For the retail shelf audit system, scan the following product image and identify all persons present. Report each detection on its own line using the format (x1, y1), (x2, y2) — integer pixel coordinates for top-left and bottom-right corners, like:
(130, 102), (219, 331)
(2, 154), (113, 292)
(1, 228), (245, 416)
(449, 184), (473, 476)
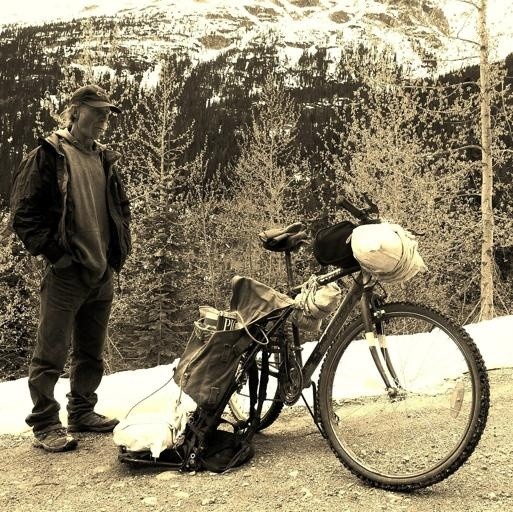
(10, 84), (131, 452)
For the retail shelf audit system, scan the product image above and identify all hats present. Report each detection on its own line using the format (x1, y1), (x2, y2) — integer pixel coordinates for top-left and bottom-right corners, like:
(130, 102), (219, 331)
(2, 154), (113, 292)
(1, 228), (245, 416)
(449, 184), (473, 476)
(70, 85), (122, 115)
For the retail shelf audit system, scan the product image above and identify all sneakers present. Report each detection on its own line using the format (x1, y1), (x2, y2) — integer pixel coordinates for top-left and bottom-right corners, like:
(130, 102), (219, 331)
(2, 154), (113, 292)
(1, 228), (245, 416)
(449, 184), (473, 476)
(67, 411), (120, 433)
(32, 427), (78, 452)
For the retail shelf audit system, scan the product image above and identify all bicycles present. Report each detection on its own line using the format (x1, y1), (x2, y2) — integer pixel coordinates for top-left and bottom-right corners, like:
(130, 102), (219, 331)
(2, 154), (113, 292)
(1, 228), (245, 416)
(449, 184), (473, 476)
(228, 192), (490, 492)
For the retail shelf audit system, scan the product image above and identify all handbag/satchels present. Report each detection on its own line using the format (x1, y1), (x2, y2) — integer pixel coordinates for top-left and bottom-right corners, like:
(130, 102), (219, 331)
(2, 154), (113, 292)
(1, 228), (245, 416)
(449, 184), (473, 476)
(170, 274), (296, 414)
